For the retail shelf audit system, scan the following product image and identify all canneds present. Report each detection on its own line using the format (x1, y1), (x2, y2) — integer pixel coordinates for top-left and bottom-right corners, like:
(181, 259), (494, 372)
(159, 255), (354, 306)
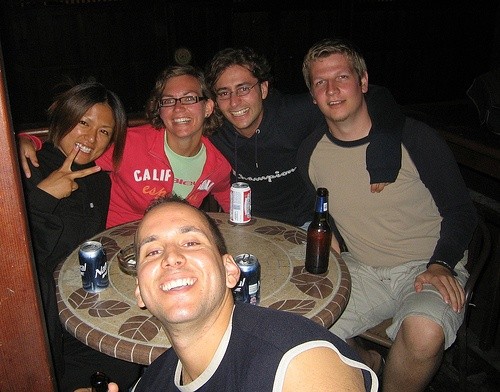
(231, 253), (260, 306)
(229, 182), (252, 223)
(78, 240), (109, 294)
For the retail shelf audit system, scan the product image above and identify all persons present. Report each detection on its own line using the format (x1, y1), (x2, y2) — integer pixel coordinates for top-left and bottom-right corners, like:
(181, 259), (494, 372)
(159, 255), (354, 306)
(22, 82), (128, 387)
(73, 193), (380, 392)
(296, 37), (476, 392)
(14, 66), (239, 229)
(206, 46), (402, 227)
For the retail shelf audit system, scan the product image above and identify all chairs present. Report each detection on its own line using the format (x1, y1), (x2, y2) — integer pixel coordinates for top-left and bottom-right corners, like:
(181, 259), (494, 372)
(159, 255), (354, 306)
(17, 115), (149, 156)
(359, 218), (488, 392)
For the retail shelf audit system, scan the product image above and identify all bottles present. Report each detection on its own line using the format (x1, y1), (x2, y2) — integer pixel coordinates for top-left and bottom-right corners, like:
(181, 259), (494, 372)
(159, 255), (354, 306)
(90, 372), (108, 392)
(305, 187), (332, 275)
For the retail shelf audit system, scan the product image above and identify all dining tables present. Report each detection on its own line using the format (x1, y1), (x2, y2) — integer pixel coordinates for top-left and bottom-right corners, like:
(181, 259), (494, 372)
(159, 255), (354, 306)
(52, 211), (352, 368)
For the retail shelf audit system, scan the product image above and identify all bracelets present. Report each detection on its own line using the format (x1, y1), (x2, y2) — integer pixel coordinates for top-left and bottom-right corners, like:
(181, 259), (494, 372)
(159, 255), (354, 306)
(421, 258), (458, 275)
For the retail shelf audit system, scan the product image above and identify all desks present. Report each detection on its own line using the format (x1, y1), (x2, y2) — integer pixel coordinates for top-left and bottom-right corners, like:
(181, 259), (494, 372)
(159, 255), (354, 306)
(420, 110), (500, 203)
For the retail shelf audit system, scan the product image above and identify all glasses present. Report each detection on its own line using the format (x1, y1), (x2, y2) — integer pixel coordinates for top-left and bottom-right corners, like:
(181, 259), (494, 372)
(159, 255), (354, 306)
(158, 96), (208, 108)
(216, 81), (259, 101)
